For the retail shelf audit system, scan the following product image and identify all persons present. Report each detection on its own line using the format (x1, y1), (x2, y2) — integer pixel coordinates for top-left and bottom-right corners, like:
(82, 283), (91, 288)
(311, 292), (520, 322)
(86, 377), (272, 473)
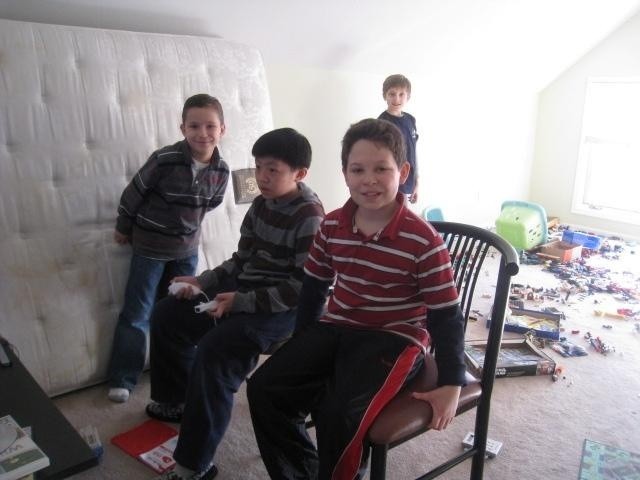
(142, 126), (328, 480)
(103, 91), (232, 403)
(245, 117), (469, 480)
(376, 72), (420, 219)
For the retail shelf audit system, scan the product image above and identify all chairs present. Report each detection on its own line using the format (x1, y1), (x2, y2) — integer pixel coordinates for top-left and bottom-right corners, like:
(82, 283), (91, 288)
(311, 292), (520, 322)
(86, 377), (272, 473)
(300, 216), (523, 480)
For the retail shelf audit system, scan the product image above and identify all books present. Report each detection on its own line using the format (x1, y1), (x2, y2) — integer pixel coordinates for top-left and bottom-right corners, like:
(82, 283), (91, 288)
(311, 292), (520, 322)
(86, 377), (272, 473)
(109, 416), (182, 475)
(579, 437), (639, 479)
(0, 413), (52, 479)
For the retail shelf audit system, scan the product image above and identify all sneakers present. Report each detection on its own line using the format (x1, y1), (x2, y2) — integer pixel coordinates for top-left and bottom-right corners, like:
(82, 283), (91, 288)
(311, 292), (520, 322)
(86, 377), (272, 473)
(147, 403), (184, 422)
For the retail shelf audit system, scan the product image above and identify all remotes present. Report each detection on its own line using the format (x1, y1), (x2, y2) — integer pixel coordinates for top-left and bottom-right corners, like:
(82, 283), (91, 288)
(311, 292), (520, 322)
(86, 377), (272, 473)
(462, 432), (502, 456)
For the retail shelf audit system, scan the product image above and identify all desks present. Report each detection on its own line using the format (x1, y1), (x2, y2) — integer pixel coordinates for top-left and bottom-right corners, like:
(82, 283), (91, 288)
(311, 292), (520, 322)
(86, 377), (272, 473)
(0, 339), (101, 479)
